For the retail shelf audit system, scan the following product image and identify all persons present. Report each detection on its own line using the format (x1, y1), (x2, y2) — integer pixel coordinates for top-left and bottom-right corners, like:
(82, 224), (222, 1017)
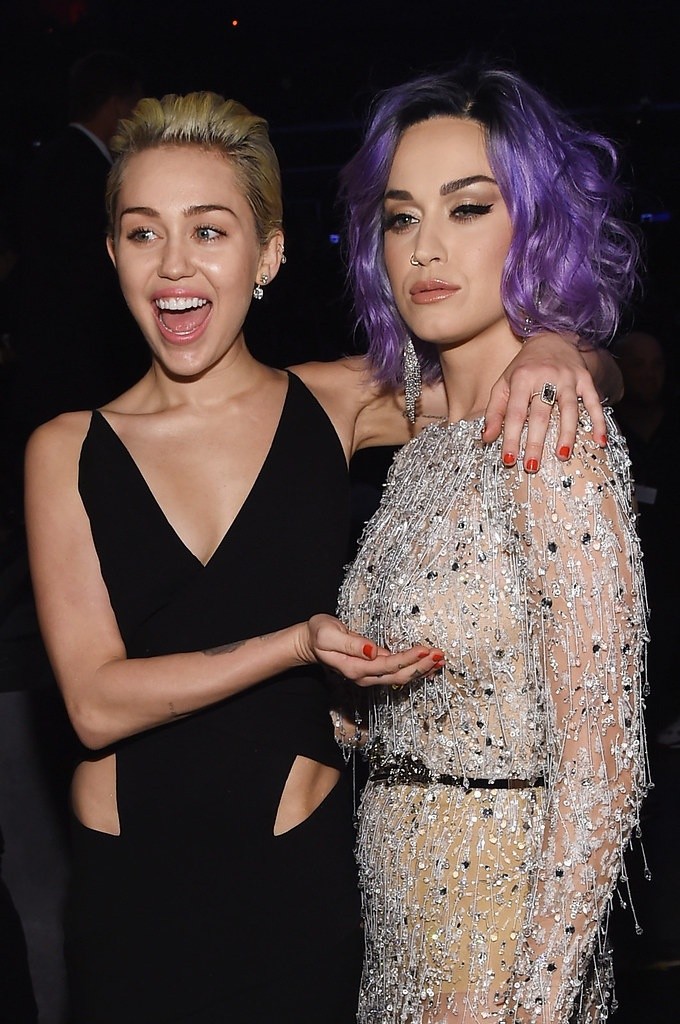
(337, 63), (648, 1024)
(24, 92), (631, 1023)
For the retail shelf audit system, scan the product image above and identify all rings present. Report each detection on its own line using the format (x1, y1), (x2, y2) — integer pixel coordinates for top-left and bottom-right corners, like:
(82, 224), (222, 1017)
(391, 683), (404, 691)
(530, 381), (557, 405)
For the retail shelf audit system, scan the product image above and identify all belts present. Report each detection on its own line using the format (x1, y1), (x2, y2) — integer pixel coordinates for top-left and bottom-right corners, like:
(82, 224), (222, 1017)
(366, 747), (550, 789)
